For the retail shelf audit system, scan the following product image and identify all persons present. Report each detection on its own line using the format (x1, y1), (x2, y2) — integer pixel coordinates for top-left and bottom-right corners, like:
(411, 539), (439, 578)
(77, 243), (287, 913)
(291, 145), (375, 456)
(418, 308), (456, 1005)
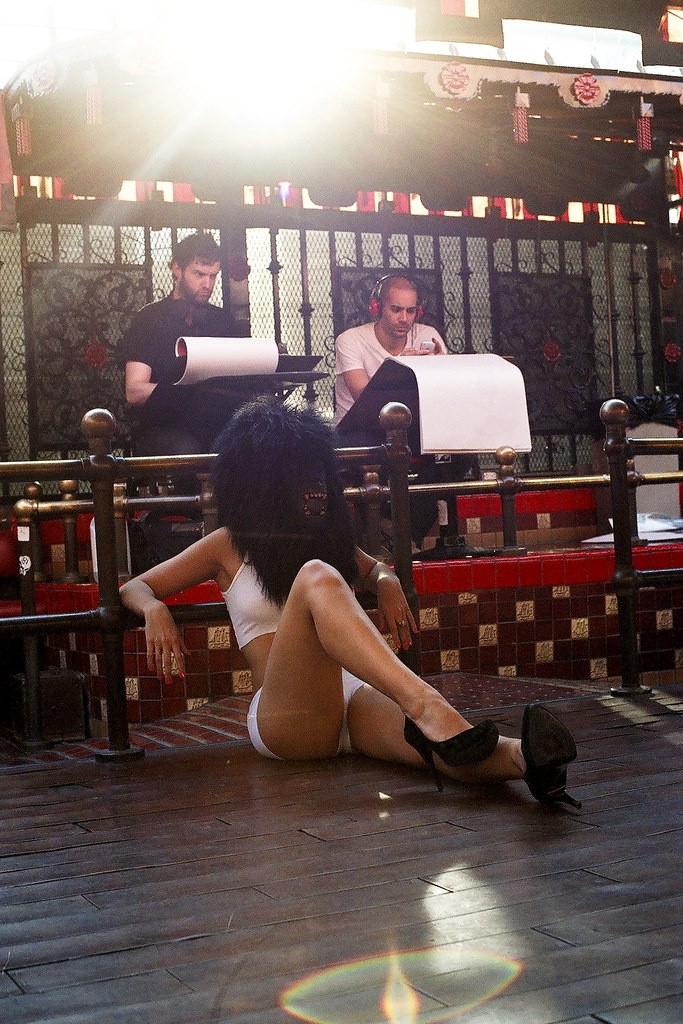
(331, 274), (479, 566)
(114, 234), (272, 482)
(115, 404), (580, 813)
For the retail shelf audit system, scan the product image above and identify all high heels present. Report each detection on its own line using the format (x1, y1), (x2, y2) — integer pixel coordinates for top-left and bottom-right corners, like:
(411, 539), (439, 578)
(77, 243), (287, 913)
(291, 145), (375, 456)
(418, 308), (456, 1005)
(402, 713), (501, 791)
(518, 699), (582, 812)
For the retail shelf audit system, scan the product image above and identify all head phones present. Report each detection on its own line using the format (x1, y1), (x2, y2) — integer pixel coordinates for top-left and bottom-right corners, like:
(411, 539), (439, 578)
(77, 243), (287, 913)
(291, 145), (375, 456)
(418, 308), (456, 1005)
(369, 274), (423, 320)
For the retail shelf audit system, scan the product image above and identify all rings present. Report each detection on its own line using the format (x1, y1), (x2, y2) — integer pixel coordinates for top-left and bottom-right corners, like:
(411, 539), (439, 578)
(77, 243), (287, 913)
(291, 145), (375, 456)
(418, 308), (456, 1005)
(397, 620), (405, 626)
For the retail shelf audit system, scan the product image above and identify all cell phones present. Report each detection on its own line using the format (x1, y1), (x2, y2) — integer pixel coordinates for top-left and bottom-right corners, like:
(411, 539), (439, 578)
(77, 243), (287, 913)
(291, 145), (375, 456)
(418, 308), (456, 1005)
(419, 342), (436, 353)
